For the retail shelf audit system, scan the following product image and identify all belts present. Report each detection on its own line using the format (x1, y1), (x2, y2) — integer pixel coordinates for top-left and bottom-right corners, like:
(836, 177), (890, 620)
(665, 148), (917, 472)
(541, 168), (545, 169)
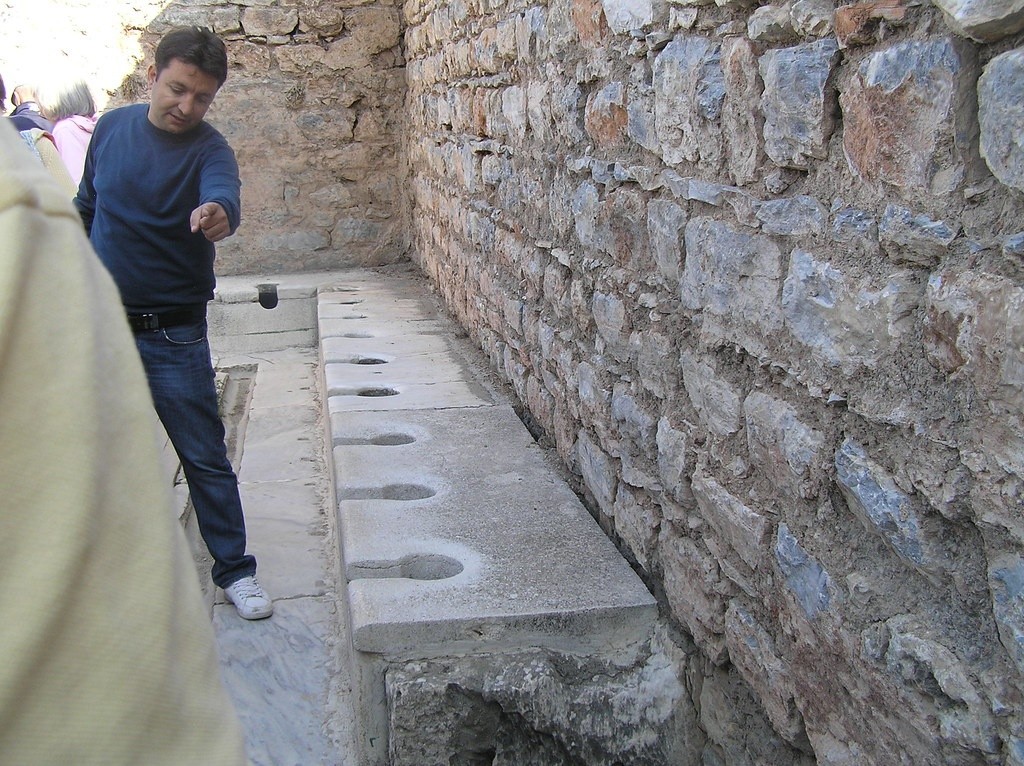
(126, 303), (207, 332)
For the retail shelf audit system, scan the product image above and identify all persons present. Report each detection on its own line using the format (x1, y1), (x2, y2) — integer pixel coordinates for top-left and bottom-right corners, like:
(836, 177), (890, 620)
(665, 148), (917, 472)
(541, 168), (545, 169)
(9, 82), (57, 134)
(0, 108), (238, 766)
(20, 127), (79, 199)
(76, 25), (272, 621)
(35, 63), (98, 188)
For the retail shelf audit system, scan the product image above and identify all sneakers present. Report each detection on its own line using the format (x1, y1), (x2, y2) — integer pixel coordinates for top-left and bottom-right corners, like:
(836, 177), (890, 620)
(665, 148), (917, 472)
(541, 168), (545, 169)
(224, 576), (273, 620)
